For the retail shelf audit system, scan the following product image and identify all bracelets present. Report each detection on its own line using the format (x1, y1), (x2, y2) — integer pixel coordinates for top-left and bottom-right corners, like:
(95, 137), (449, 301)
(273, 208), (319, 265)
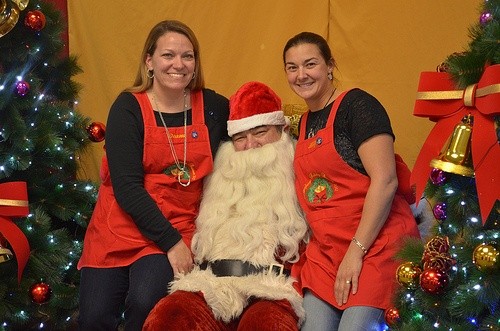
(352, 238), (367, 255)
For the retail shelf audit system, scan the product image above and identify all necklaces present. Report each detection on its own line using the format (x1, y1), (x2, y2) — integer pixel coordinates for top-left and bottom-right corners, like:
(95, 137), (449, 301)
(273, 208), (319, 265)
(151, 90), (191, 188)
(308, 89), (336, 140)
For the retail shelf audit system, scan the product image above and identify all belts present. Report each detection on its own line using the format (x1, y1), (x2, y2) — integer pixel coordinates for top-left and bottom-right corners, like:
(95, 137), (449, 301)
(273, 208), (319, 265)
(199, 259), (290, 277)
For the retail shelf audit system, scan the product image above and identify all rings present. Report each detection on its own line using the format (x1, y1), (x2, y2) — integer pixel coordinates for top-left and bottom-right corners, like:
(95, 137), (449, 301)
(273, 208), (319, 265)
(346, 281), (350, 283)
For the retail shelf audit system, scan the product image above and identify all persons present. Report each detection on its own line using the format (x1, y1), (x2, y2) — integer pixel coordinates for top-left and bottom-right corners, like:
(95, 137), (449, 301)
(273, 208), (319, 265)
(142, 83), (423, 331)
(283, 32), (421, 331)
(75, 20), (289, 331)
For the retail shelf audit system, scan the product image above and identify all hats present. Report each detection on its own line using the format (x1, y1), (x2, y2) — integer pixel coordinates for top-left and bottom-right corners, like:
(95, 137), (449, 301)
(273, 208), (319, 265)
(227, 81), (291, 137)
